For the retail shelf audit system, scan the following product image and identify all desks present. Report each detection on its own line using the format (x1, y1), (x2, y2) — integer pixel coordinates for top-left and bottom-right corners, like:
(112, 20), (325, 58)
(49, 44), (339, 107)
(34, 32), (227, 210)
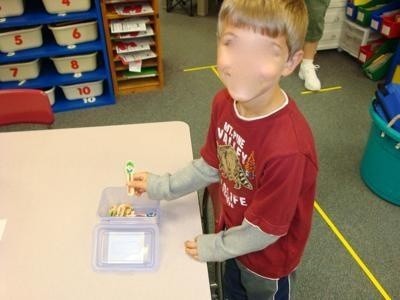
(2, 120), (214, 300)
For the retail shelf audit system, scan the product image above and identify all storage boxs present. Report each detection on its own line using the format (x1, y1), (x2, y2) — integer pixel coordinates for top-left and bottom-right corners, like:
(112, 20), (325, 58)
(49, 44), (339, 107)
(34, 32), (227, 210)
(90, 183), (164, 274)
(1, 1), (106, 109)
(340, 0), (400, 81)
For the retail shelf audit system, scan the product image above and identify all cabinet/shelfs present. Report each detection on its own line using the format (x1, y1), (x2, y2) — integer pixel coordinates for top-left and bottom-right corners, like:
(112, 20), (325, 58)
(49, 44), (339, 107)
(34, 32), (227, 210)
(1, 0), (116, 114)
(101, 0), (166, 96)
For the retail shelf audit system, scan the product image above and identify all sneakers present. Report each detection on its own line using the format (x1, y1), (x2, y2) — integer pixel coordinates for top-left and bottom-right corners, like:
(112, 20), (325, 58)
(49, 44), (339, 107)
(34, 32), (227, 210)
(298, 62), (321, 91)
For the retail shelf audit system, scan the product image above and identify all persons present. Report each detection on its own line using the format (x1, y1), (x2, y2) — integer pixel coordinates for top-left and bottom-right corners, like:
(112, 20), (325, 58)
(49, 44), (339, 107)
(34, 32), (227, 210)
(296, 0), (330, 93)
(127, 0), (320, 300)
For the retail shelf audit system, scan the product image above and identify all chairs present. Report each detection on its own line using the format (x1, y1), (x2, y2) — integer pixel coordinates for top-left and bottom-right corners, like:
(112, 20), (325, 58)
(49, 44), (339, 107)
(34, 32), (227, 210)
(1, 89), (57, 130)
